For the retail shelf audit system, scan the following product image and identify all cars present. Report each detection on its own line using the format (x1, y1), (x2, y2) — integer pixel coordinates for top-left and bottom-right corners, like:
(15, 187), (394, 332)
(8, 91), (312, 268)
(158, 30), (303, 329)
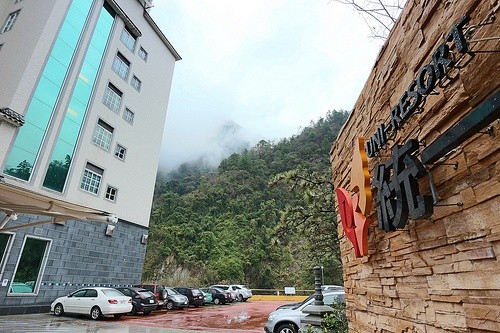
(200, 290), (213, 305)
(264, 292), (345, 333)
(164, 288), (189, 311)
(115, 287), (158, 316)
(233, 285), (252, 302)
(51, 286), (133, 321)
(10, 282), (34, 293)
(276, 285), (344, 311)
(205, 287), (232, 305)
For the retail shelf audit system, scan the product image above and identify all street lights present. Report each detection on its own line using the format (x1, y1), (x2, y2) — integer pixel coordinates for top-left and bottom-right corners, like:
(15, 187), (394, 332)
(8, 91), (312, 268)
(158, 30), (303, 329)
(322, 265), (324, 285)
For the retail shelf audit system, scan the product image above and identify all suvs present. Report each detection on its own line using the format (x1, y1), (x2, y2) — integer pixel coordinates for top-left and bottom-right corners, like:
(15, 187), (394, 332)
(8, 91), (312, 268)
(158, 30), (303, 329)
(211, 285), (237, 302)
(173, 287), (204, 307)
(132, 284), (167, 310)
